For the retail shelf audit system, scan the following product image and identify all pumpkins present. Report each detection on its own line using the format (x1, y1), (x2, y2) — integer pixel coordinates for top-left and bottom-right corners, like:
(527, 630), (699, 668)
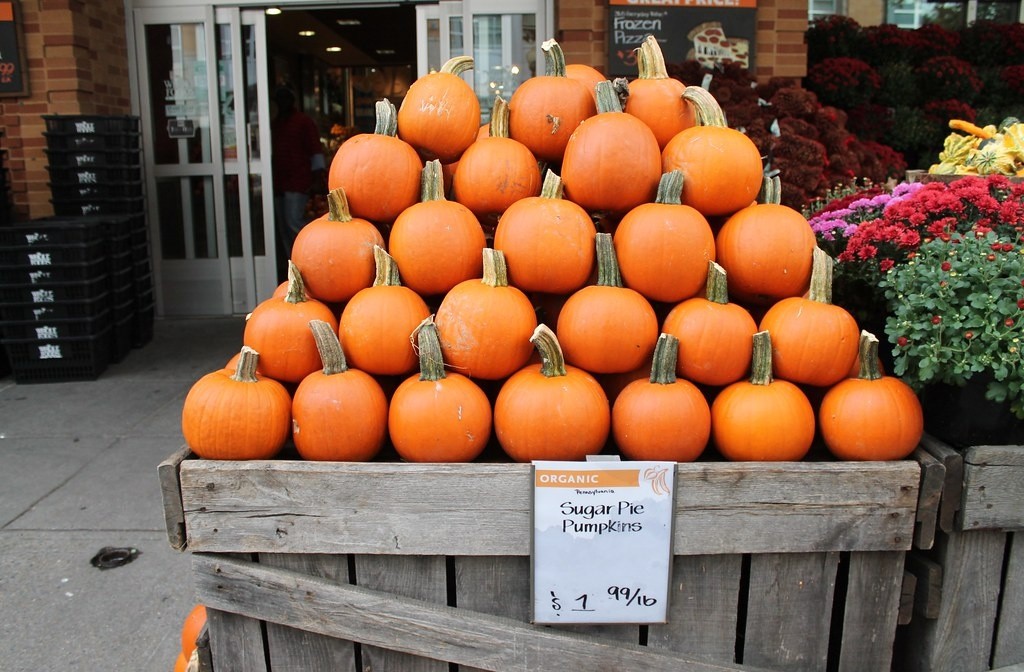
(175, 652), (187, 672)
(182, 36), (924, 459)
(182, 606), (207, 662)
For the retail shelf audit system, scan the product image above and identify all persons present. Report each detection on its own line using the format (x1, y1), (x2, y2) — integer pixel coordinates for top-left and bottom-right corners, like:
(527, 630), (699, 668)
(271, 86), (325, 258)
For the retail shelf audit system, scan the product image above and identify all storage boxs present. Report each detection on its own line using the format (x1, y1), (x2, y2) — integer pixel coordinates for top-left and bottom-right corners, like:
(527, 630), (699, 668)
(0, 114), (156, 385)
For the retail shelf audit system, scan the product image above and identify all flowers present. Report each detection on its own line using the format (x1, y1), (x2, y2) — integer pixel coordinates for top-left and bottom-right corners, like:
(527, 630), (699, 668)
(669, 13), (1024, 448)
